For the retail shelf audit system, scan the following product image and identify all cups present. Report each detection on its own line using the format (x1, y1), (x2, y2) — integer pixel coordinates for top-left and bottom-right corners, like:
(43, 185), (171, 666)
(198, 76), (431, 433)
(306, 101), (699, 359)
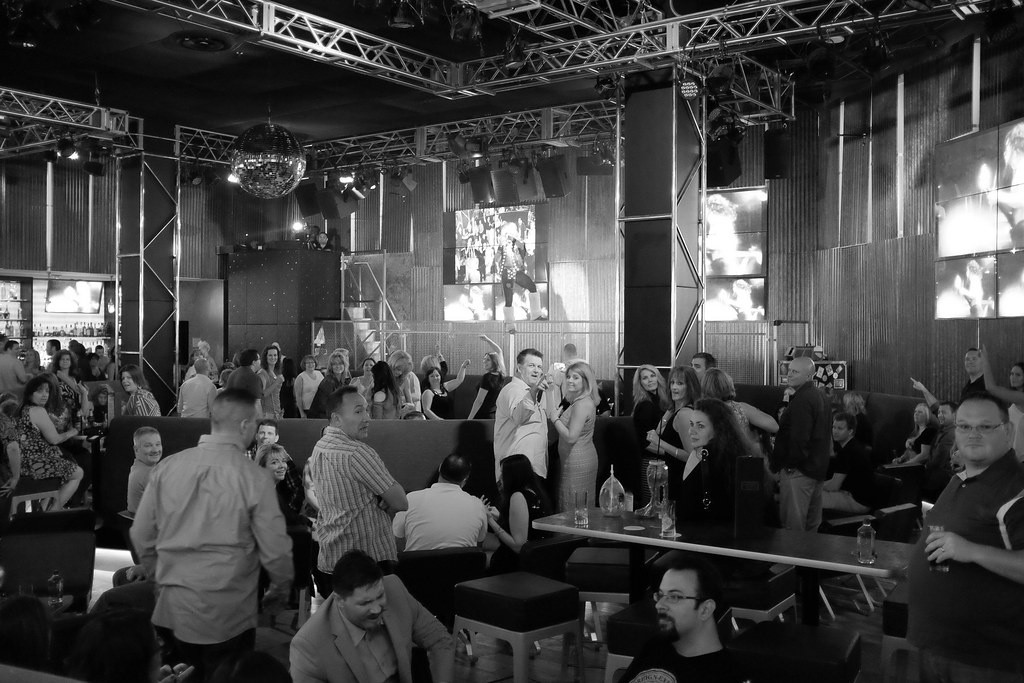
(574, 489), (589, 525)
(926, 525), (950, 574)
(661, 499), (675, 537)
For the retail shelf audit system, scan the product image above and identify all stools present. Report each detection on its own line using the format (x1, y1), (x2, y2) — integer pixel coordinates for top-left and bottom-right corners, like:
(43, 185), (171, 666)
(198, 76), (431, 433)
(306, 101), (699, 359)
(451, 571), (585, 682)
(604, 602), (864, 683)
(880, 581), (922, 683)
(8, 476), (62, 518)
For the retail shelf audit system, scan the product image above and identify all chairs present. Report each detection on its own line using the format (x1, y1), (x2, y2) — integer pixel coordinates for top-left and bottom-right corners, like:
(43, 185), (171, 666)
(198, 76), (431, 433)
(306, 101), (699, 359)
(764, 462), (926, 619)
(118, 508), (645, 631)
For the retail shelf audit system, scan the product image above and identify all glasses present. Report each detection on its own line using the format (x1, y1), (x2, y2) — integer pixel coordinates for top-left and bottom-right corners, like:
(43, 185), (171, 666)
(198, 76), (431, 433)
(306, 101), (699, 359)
(953, 422), (1007, 434)
(653, 592), (706, 604)
(255, 356), (263, 361)
(152, 637), (171, 657)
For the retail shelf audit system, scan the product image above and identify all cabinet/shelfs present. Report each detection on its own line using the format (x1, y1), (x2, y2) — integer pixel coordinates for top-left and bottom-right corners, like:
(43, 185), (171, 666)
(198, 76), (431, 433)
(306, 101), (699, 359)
(0, 277), (33, 350)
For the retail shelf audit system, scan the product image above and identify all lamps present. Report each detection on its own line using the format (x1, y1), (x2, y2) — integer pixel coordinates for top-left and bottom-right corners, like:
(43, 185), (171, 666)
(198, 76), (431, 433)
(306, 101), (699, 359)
(292, 166), (418, 221)
(675, 26), (944, 189)
(456, 134), (616, 205)
(3, 125), (221, 191)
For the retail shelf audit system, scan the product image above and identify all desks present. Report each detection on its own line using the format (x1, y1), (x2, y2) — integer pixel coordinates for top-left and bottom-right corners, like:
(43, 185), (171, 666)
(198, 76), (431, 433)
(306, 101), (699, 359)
(531, 505), (915, 626)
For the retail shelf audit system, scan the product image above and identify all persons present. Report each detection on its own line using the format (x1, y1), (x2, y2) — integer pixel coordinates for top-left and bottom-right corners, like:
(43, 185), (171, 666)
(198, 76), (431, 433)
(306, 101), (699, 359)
(118, 340), (1024, 578)
(296, 225), (321, 248)
(289, 548), (456, 683)
(0, 596), (293, 683)
(619, 553), (751, 683)
(0, 333), (121, 519)
(313, 232), (334, 249)
(303, 424), (335, 620)
(718, 279), (766, 321)
(127, 426), (165, 515)
(700, 194), (761, 276)
(490, 222), (547, 333)
(392, 453), (487, 552)
(310, 385), (408, 607)
(906, 394), (1024, 683)
(113, 388), (305, 683)
(453, 208), (533, 279)
(954, 260), (984, 317)
(986, 122), (1024, 247)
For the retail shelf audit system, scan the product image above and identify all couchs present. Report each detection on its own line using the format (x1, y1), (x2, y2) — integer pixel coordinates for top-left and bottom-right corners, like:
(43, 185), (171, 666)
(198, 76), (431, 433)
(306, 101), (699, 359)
(0, 378), (149, 407)
(0, 508), (96, 613)
(102, 370), (640, 561)
(733, 382), (959, 462)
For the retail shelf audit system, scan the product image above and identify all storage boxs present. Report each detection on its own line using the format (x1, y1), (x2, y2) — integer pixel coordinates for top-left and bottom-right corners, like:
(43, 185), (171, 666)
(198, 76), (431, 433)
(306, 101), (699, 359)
(227, 249), (341, 382)
(777, 360), (848, 392)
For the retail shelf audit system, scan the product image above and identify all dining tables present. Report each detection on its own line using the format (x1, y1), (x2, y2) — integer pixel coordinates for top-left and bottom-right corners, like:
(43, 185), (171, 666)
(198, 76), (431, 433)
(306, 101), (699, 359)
(68, 435), (106, 508)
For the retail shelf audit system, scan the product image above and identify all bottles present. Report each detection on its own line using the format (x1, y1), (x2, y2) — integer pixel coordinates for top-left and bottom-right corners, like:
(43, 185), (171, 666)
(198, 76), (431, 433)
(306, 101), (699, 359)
(857, 517), (876, 564)
(47, 571), (62, 606)
(646, 460), (668, 517)
(598, 471), (626, 517)
(87, 409), (95, 429)
(72, 410), (83, 436)
